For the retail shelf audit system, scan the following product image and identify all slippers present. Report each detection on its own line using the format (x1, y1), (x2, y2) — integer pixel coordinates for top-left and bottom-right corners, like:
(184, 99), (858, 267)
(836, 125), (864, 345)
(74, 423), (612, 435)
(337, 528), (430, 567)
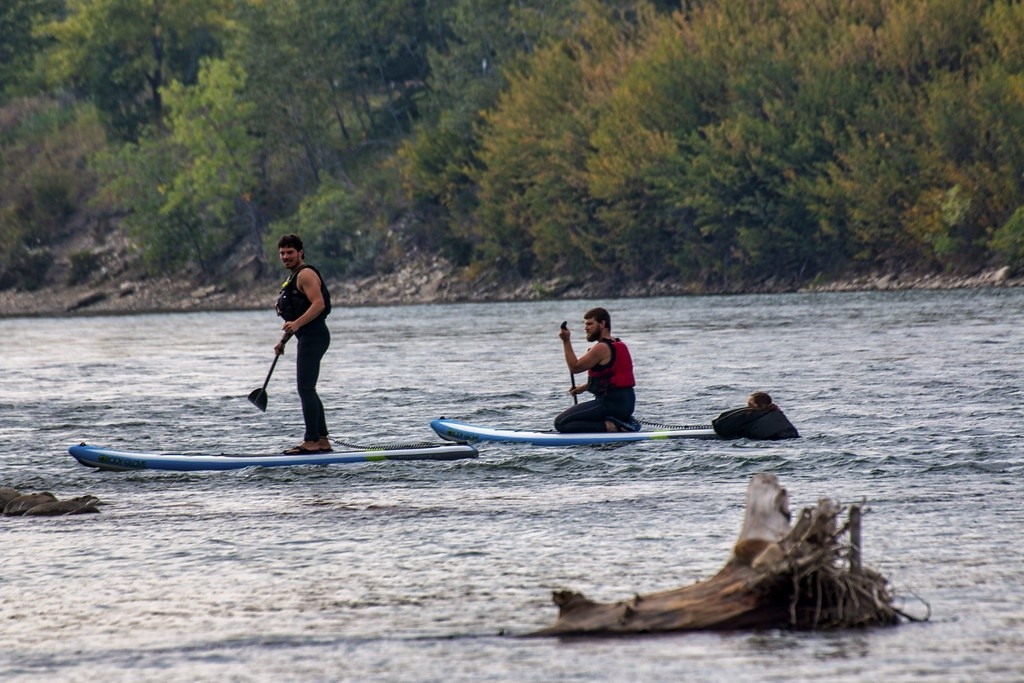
(604, 415), (641, 432)
(283, 446), (334, 455)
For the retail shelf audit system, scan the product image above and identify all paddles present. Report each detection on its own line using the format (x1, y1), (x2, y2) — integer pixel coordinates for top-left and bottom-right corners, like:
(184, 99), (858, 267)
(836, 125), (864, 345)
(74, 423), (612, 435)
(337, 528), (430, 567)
(248, 340), (288, 413)
(561, 321), (579, 405)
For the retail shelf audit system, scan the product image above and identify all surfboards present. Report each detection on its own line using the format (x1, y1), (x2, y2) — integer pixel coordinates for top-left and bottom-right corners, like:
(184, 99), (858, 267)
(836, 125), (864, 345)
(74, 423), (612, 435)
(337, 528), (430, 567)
(431, 419), (717, 445)
(68, 445), (478, 470)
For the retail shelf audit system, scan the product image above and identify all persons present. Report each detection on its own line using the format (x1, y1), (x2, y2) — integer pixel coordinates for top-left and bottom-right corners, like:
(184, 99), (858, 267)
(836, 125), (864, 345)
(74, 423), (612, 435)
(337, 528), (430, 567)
(274, 233), (333, 457)
(554, 308), (641, 436)
(744, 390), (775, 407)
